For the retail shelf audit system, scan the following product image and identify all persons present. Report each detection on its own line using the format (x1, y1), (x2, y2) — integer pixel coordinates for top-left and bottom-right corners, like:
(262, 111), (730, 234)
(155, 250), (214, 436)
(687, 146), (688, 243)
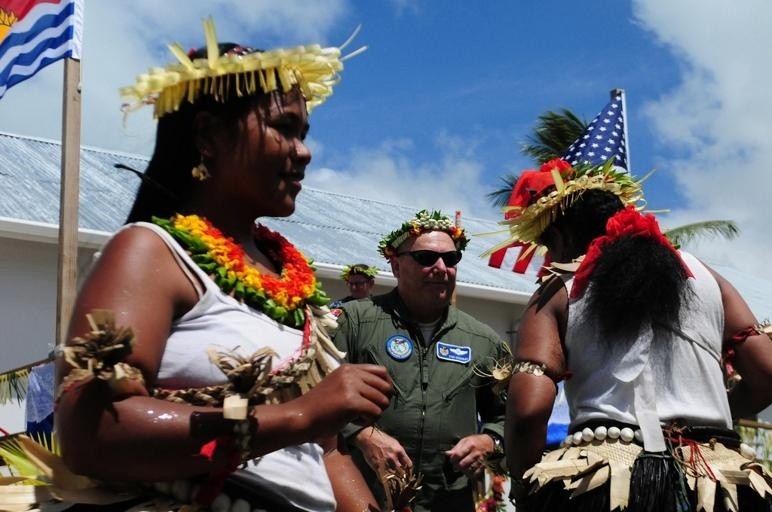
(502, 183), (772, 510)
(53, 41), (397, 511)
(324, 210), (516, 512)
(327, 263), (376, 309)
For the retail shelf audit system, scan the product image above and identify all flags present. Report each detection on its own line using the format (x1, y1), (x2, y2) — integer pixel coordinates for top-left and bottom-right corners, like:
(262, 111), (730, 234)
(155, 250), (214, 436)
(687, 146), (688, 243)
(559, 92), (626, 175)
(1, 0), (82, 99)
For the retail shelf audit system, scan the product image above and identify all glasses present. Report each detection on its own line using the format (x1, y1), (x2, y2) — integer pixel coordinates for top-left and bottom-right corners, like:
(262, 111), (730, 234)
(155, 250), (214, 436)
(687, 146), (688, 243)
(396, 246), (462, 267)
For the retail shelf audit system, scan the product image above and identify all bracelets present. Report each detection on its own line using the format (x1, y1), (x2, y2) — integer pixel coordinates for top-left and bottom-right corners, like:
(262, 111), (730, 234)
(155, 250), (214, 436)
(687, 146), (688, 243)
(489, 431), (503, 454)
